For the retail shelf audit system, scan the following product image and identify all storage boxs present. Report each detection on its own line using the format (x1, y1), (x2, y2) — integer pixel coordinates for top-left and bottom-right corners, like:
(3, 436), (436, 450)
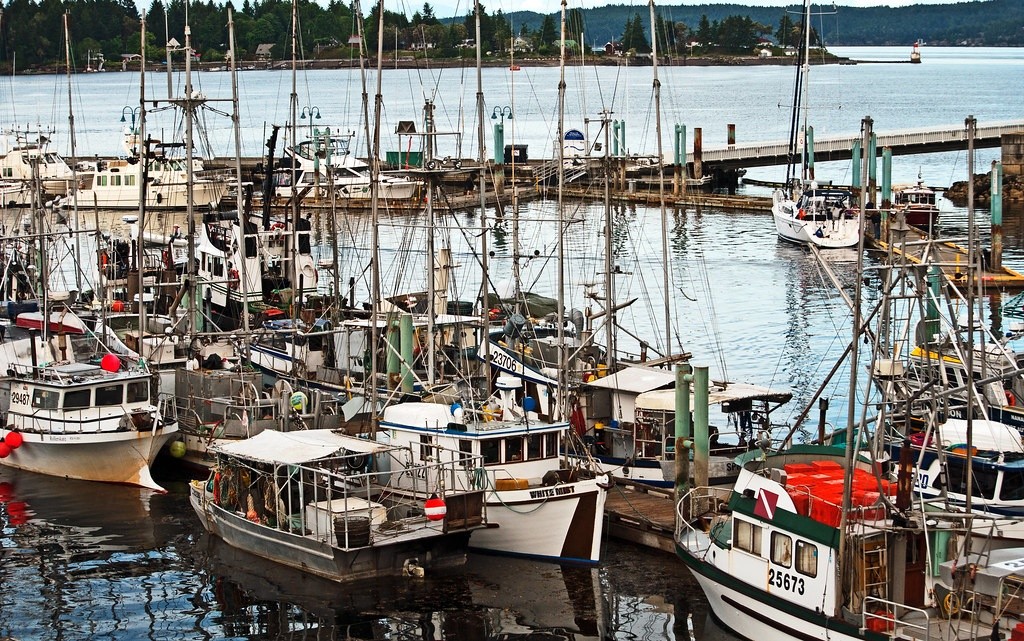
(138, 338), (175, 361)
(306, 497), (387, 535)
(149, 318), (171, 334)
(951, 444), (977, 456)
(783, 459), (897, 528)
(864, 609), (894, 631)
(910, 433), (932, 446)
(126, 330), (152, 353)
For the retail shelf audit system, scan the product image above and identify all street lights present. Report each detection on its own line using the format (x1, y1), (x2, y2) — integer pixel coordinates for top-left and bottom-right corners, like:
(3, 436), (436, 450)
(119, 105), (144, 131)
(299, 106), (322, 139)
(491, 106), (515, 164)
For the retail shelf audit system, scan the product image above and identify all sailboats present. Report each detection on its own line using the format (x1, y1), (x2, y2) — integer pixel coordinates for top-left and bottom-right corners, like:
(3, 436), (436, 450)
(0, 0), (1024, 554)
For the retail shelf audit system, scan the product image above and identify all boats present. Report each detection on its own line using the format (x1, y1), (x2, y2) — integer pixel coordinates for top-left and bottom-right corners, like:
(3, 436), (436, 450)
(187, 425), (501, 584)
(671, 219), (1023, 641)
